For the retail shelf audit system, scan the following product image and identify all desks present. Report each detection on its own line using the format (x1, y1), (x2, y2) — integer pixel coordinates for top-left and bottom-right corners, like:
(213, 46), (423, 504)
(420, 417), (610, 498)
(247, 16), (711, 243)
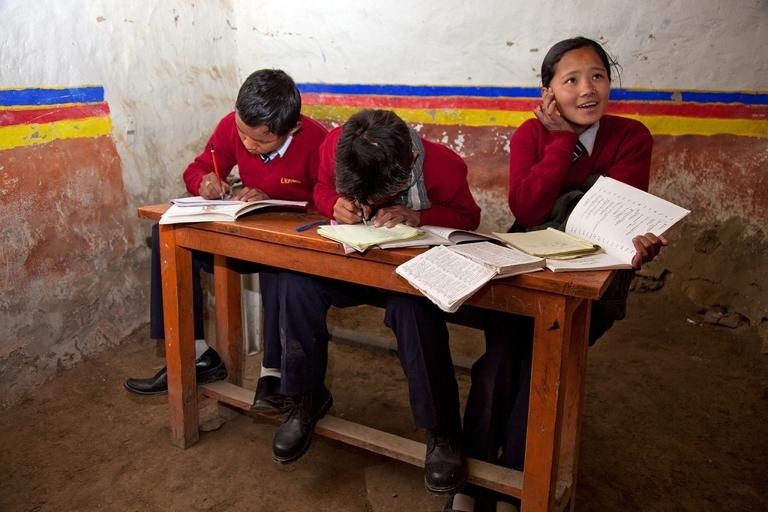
(138, 203), (615, 512)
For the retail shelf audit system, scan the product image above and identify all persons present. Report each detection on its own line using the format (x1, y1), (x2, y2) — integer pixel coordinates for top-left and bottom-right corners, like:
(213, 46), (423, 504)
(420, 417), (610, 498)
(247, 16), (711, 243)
(444, 35), (671, 511)
(273, 107), (470, 490)
(122, 67), (329, 414)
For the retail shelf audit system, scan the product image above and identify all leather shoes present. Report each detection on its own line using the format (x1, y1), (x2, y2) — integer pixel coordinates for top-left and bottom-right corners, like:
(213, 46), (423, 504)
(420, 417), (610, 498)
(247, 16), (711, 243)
(250, 376), (287, 414)
(423, 421), (469, 495)
(271, 382), (333, 464)
(124, 346), (228, 396)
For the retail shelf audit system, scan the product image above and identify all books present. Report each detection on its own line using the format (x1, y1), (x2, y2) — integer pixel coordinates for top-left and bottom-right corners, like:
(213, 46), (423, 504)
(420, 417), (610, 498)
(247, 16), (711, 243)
(169, 196), (244, 206)
(492, 225), (601, 260)
(543, 173), (693, 275)
(154, 198), (308, 226)
(368, 245), (430, 251)
(377, 224), (503, 253)
(330, 219), (380, 255)
(315, 219), (427, 253)
(395, 240), (546, 314)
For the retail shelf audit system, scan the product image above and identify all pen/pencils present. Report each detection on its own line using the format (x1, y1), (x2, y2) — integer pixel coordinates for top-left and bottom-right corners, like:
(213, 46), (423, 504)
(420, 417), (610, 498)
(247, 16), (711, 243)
(210, 144), (224, 200)
(354, 196), (367, 225)
(296, 220), (326, 231)
(539, 103), (543, 112)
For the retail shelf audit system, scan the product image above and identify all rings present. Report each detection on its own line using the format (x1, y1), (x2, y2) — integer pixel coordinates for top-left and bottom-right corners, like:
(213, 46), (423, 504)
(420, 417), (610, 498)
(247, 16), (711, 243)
(205, 181), (212, 187)
